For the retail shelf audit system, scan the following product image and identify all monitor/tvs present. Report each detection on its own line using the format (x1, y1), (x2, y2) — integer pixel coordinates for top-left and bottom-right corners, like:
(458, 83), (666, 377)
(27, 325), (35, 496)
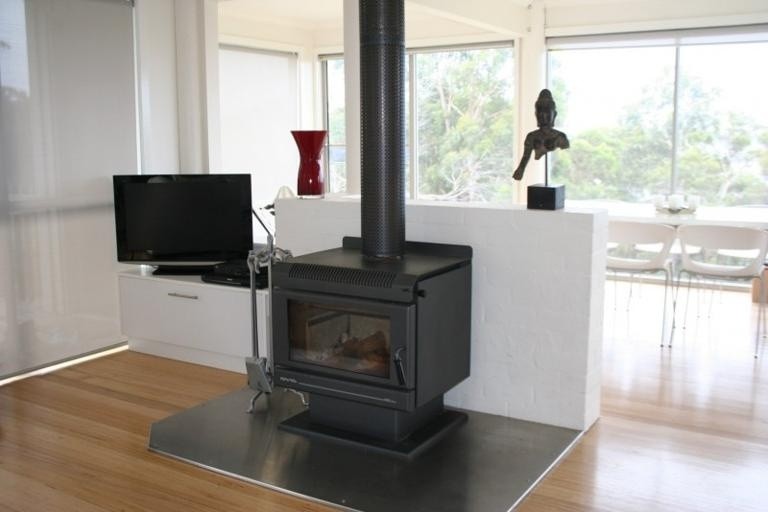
(113, 174), (253, 275)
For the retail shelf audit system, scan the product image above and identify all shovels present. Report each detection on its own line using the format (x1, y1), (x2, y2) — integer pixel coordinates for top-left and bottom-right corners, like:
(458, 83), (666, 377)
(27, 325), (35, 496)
(245, 255), (273, 393)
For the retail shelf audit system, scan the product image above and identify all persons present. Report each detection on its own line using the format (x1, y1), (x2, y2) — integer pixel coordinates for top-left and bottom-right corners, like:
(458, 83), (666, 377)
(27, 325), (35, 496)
(513, 89), (570, 181)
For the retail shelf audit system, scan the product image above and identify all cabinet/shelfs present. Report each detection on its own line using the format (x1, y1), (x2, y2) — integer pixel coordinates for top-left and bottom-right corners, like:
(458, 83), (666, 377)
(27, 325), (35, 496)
(118, 267), (269, 374)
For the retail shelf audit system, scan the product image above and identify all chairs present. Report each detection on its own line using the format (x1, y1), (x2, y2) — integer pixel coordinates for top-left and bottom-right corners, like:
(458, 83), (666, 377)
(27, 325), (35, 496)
(564, 197), (768, 359)
